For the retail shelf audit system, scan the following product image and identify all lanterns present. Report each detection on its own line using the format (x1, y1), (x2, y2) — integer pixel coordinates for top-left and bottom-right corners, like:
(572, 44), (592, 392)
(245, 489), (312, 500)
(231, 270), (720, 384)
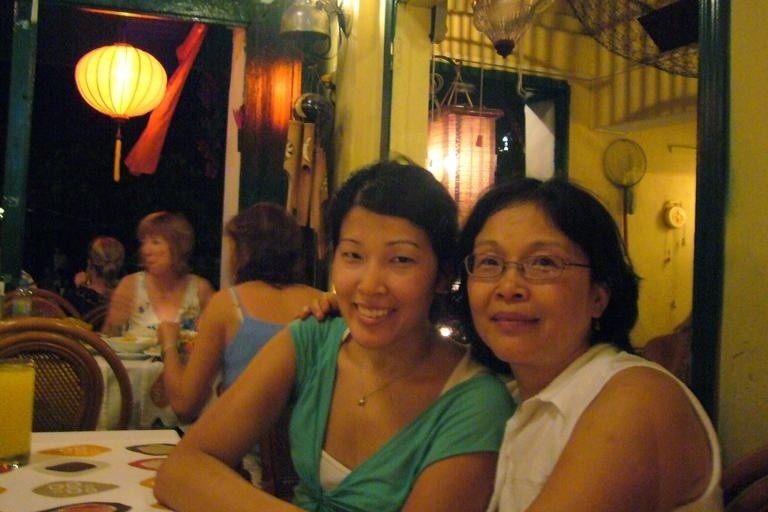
(73, 42), (168, 183)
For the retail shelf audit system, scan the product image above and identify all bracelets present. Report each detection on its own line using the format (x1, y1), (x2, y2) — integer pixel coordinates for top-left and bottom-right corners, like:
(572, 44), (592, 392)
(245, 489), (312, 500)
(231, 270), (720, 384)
(162, 342), (177, 353)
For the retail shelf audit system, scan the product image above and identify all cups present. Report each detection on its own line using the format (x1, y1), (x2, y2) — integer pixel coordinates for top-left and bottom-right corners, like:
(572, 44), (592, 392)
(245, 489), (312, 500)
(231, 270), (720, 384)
(0, 359), (39, 468)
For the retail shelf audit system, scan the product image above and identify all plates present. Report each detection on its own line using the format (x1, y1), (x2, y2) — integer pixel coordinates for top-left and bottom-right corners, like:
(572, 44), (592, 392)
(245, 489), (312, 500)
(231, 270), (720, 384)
(115, 352), (150, 361)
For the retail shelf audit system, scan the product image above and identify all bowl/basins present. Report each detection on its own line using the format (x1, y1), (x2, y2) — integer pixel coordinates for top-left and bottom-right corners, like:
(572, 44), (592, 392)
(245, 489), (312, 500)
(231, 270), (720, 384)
(105, 336), (156, 353)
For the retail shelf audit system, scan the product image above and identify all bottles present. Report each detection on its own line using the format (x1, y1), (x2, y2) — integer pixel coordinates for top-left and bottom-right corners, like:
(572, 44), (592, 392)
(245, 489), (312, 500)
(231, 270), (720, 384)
(11, 279), (33, 320)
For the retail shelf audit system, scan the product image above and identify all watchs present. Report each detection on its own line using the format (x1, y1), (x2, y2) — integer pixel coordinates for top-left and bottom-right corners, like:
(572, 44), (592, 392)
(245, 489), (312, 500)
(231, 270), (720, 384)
(148, 149), (517, 512)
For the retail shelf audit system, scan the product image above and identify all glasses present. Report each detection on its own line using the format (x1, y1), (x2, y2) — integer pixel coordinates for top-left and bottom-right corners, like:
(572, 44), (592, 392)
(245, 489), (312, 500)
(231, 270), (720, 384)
(464, 253), (591, 281)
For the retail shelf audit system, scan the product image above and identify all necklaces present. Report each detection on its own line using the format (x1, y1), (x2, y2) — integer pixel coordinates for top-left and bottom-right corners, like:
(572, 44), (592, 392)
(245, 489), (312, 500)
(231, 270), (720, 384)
(350, 337), (431, 410)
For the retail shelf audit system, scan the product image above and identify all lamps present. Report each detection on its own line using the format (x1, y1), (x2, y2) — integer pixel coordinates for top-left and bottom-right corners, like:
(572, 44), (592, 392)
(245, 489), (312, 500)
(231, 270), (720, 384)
(473, 1), (535, 59)
(278, 0), (353, 45)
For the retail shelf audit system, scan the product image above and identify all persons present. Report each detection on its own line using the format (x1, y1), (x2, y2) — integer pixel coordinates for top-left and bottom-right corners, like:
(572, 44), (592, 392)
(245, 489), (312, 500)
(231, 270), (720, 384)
(100, 209), (218, 339)
(64, 238), (126, 333)
(157, 198), (331, 484)
(454, 175), (726, 509)
